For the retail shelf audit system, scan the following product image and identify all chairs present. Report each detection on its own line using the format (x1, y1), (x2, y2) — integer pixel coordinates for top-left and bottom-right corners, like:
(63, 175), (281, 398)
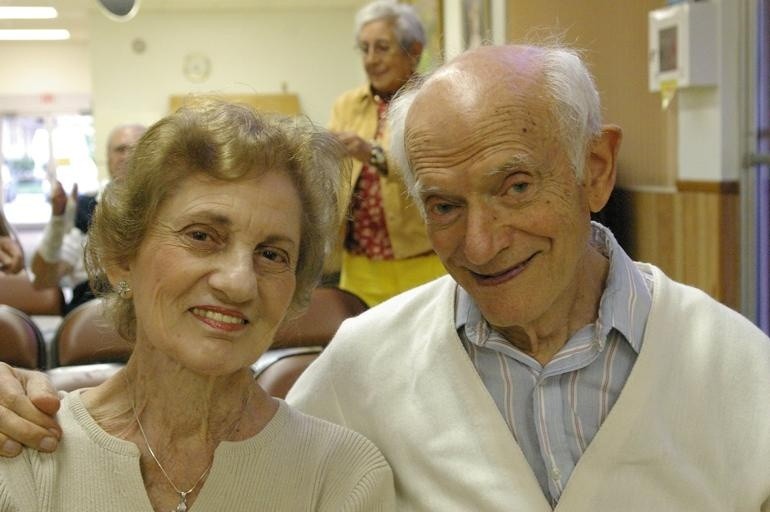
(269, 283), (372, 348)
(52, 290), (138, 364)
(251, 351), (323, 402)
(0, 265), (65, 316)
(1, 302), (47, 373)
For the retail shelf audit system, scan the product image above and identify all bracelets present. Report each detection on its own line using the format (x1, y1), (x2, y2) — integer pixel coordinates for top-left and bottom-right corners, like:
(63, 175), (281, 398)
(370, 140), (384, 169)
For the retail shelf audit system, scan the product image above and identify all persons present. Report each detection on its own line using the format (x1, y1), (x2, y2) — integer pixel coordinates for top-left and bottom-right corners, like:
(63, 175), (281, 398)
(0, 233), (28, 276)
(0, 42), (770, 511)
(327, 0), (451, 310)
(31, 122), (148, 322)
(0, 90), (396, 512)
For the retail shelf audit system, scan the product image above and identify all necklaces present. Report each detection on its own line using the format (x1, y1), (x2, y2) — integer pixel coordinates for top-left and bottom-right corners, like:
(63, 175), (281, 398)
(123, 365), (249, 512)
